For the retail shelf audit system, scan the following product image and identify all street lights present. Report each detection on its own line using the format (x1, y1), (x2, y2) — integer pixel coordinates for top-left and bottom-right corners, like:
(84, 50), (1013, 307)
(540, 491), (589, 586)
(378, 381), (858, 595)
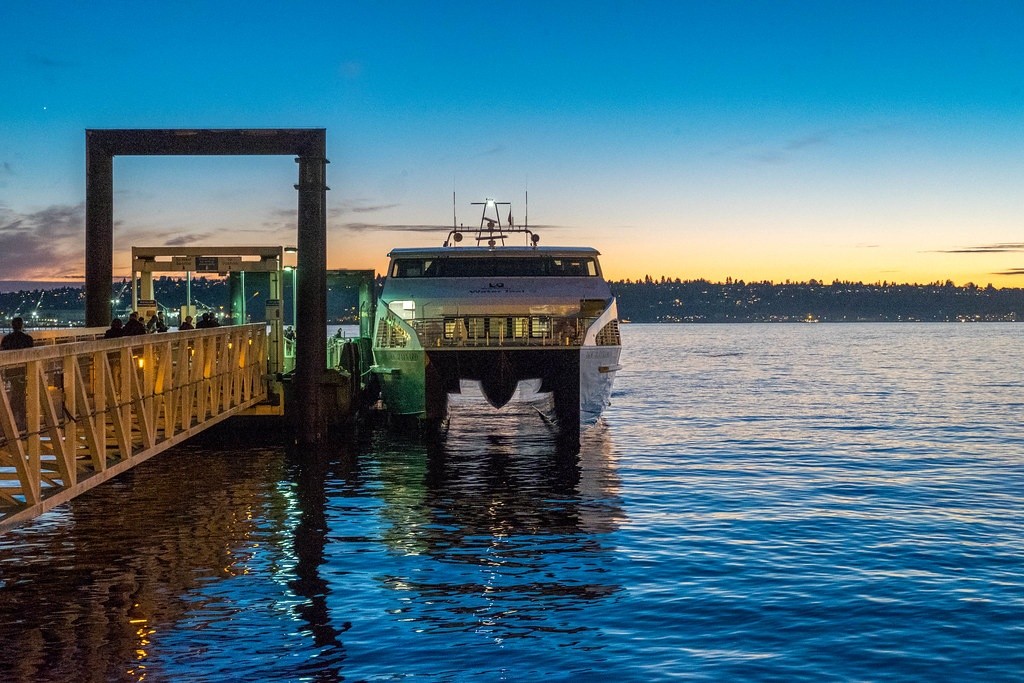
(283, 266), (296, 337)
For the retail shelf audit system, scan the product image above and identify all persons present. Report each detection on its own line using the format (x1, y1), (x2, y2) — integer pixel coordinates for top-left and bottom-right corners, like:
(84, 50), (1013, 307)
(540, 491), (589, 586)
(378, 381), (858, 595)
(283, 325), (297, 356)
(104, 311), (221, 371)
(0, 317), (33, 432)
(332, 327), (343, 339)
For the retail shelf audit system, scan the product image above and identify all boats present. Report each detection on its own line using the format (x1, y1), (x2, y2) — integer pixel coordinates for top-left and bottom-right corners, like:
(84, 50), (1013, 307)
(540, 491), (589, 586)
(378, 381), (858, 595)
(371, 174), (622, 434)
